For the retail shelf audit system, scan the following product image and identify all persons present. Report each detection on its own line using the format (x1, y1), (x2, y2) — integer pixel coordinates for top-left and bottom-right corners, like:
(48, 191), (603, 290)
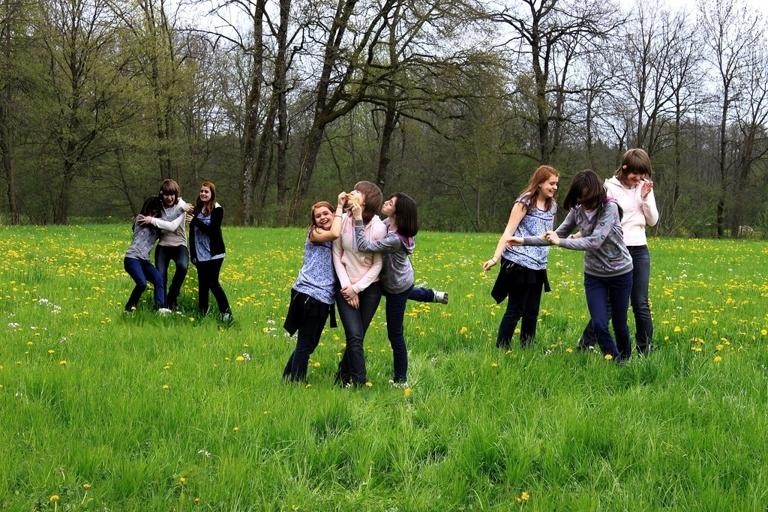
(124, 196), (194, 314)
(483, 165), (560, 350)
(351, 192), (448, 388)
(576, 149), (659, 356)
(282, 192), (347, 384)
(505, 170), (634, 364)
(332, 181), (380, 391)
(138, 179), (189, 307)
(185, 182), (233, 329)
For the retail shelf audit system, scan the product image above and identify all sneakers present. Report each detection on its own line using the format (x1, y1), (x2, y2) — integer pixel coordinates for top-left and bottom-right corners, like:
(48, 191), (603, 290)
(158, 307), (172, 313)
(435, 291), (449, 304)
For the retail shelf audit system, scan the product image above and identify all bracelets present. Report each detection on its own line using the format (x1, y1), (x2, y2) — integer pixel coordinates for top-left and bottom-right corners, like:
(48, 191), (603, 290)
(337, 206), (343, 210)
(334, 215), (342, 218)
(490, 258), (496, 265)
